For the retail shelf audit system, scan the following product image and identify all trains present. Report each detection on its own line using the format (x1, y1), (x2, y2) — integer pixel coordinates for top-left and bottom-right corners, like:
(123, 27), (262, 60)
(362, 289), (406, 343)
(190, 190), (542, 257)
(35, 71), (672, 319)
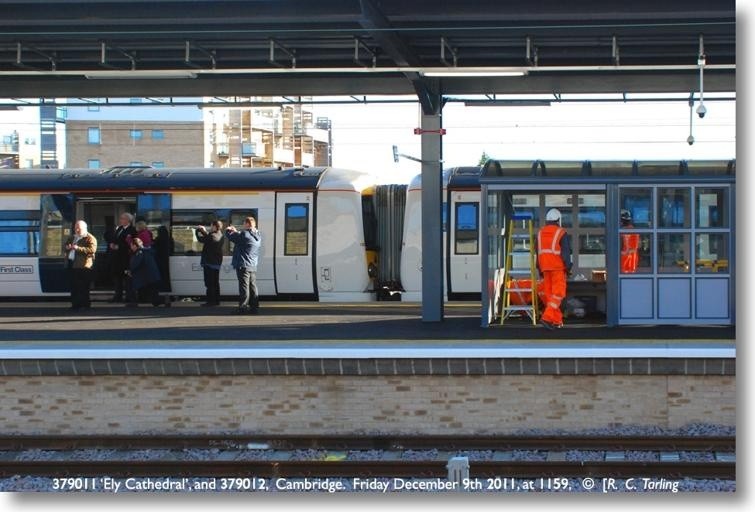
(1, 154), (734, 319)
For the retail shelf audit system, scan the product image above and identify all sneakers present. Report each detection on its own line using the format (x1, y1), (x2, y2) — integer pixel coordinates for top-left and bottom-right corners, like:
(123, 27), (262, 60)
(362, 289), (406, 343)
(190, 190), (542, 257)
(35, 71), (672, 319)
(539, 319), (555, 330)
(231, 308), (260, 315)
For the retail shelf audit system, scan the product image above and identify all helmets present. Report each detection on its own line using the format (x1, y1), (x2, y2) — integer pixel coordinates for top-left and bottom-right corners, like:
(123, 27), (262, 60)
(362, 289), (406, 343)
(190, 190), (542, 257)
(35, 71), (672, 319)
(621, 209), (632, 221)
(546, 208), (561, 222)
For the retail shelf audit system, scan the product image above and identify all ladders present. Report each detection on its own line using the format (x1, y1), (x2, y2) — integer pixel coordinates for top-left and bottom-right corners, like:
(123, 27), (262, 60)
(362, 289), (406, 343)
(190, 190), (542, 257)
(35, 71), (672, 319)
(502, 210), (539, 325)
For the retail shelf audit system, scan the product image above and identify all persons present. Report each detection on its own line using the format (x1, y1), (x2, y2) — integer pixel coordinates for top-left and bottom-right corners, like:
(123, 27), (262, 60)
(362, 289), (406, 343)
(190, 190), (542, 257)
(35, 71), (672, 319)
(620, 209), (640, 274)
(63, 220), (97, 308)
(195, 220), (224, 307)
(224, 216), (262, 315)
(536, 207), (572, 331)
(104, 211), (174, 309)
